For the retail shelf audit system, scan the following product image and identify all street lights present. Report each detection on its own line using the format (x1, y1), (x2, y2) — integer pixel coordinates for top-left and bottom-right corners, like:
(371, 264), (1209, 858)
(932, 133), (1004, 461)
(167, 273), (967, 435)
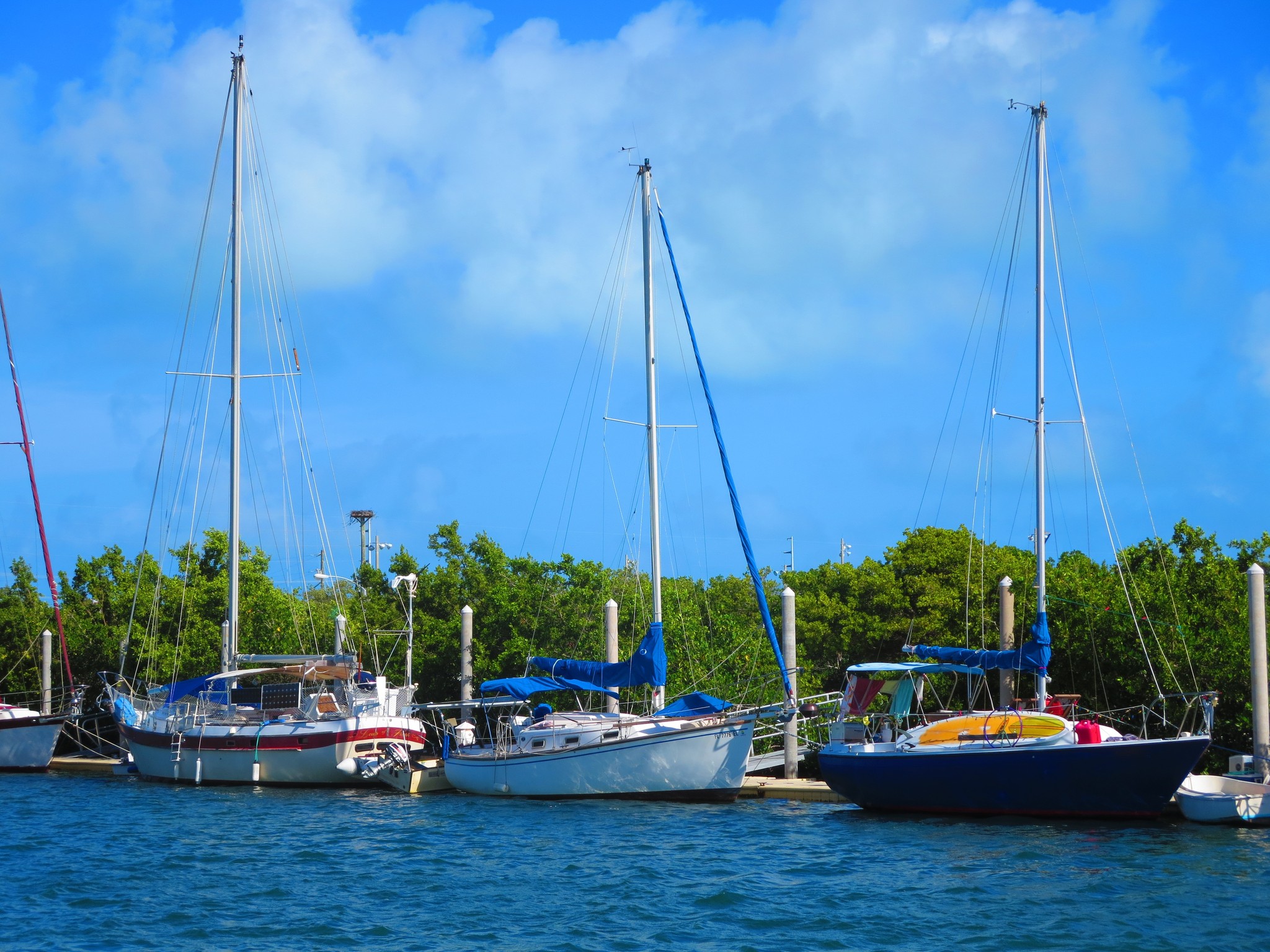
(312, 573), (367, 599)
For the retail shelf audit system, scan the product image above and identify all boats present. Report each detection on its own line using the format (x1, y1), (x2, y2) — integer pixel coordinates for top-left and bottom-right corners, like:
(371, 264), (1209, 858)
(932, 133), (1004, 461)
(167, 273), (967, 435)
(1173, 774), (1269, 827)
(378, 743), (461, 794)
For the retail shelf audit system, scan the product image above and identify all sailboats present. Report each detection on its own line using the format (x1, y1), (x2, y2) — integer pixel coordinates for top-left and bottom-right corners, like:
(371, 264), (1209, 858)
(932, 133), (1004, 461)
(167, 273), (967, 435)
(812, 97), (1223, 825)
(403, 157), (843, 800)
(0, 286), (82, 772)
(93, 29), (432, 792)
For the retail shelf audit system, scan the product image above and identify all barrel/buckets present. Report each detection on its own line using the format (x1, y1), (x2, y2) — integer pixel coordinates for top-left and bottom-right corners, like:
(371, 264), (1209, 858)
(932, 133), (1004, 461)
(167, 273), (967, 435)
(1074, 720), (1101, 745)
